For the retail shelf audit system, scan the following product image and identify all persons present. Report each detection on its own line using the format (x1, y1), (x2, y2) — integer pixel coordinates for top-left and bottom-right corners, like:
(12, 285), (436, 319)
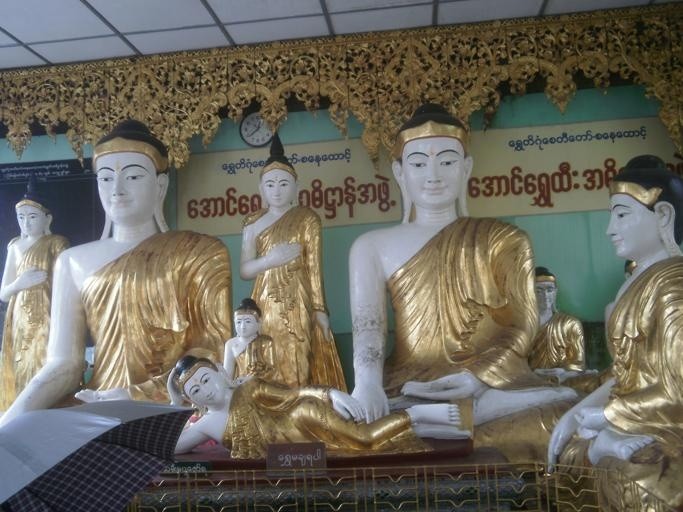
(155, 352), (474, 455)
(534, 142), (681, 508)
(598, 257), (653, 366)
(239, 132), (346, 395)
(527, 259), (588, 374)
(1, 116), (233, 424)
(344, 96), (542, 411)
(217, 295), (272, 379)
(1, 172), (84, 417)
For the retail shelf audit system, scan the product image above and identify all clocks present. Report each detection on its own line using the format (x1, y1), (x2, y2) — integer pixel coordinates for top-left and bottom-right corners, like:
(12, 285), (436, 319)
(239, 110), (276, 147)
